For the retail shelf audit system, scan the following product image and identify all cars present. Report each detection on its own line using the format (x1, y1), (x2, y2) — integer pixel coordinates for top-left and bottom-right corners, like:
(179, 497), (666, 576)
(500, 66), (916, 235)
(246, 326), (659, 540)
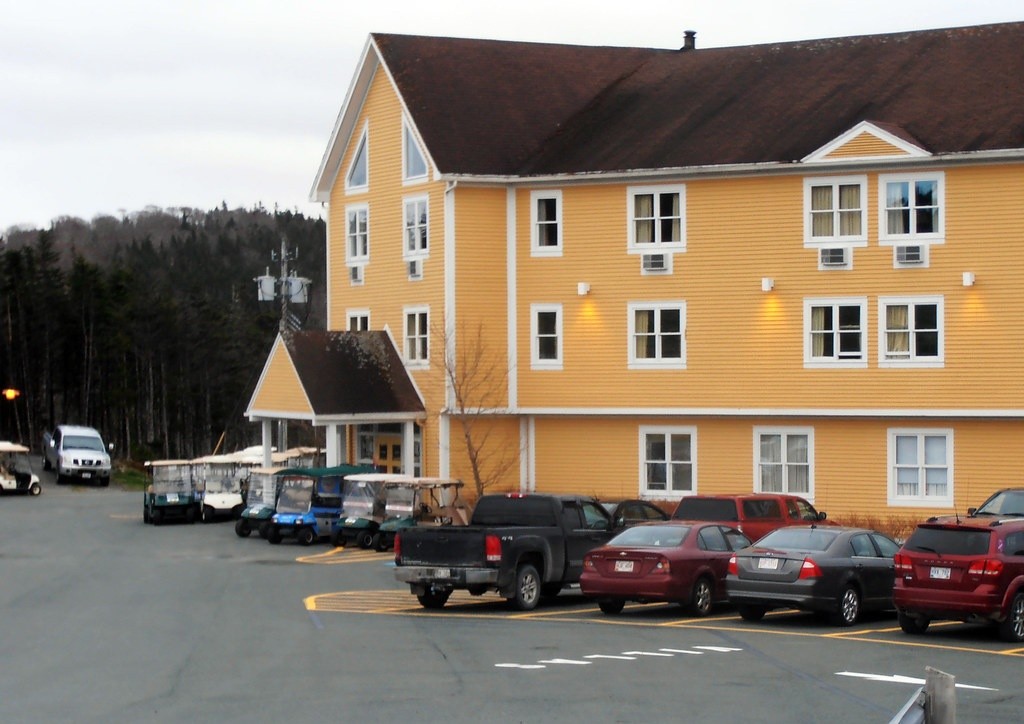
(595, 498), (672, 529)
(582, 519), (758, 619)
(726, 525), (901, 628)
(967, 487), (1024, 516)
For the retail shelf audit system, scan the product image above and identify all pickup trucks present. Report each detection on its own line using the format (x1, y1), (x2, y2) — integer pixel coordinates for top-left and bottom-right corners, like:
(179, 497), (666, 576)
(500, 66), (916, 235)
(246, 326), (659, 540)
(41, 425), (114, 486)
(392, 493), (627, 613)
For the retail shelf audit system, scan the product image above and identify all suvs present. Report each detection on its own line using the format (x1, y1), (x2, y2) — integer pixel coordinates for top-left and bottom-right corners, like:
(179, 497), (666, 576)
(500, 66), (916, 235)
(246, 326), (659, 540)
(891, 512), (1024, 643)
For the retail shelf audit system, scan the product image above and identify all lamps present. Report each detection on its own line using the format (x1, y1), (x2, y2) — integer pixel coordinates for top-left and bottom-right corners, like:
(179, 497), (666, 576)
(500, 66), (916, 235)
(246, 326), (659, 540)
(577, 282), (591, 295)
(963, 272), (975, 287)
(762, 277), (775, 291)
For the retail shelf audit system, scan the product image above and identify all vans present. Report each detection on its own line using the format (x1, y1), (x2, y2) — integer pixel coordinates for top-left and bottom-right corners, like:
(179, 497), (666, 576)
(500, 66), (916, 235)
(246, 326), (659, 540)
(671, 494), (841, 545)
